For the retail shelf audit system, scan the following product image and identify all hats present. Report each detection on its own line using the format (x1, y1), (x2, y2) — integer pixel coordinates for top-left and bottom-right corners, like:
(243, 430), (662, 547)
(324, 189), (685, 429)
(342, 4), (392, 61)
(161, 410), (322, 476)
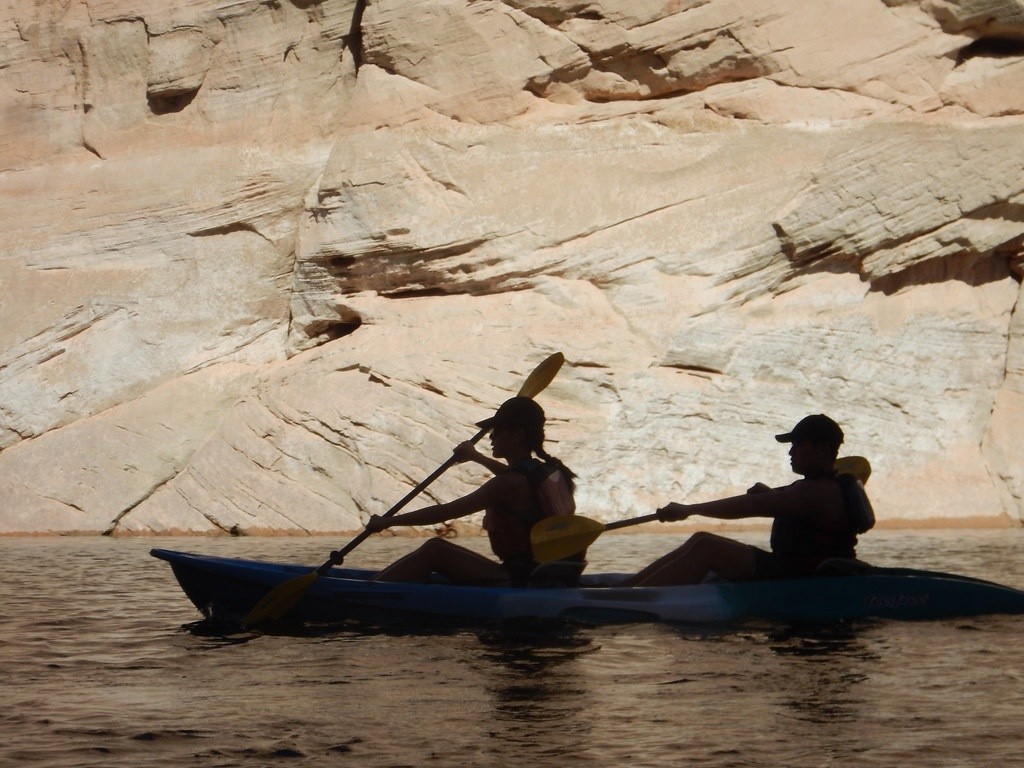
(475, 396), (546, 429)
(776, 414), (844, 445)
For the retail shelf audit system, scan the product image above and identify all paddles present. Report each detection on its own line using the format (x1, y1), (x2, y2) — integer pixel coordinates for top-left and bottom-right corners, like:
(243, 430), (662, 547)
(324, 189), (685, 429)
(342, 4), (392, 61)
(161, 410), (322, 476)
(529, 456), (872, 565)
(242, 353), (565, 633)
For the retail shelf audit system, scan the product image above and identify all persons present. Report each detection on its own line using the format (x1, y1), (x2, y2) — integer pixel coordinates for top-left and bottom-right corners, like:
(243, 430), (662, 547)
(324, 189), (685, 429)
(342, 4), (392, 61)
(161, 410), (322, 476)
(594, 414), (857, 588)
(364, 396), (587, 588)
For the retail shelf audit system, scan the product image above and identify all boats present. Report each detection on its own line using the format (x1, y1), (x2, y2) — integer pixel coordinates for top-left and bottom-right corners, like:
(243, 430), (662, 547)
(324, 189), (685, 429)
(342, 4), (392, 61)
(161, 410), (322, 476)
(147, 546), (1024, 638)
